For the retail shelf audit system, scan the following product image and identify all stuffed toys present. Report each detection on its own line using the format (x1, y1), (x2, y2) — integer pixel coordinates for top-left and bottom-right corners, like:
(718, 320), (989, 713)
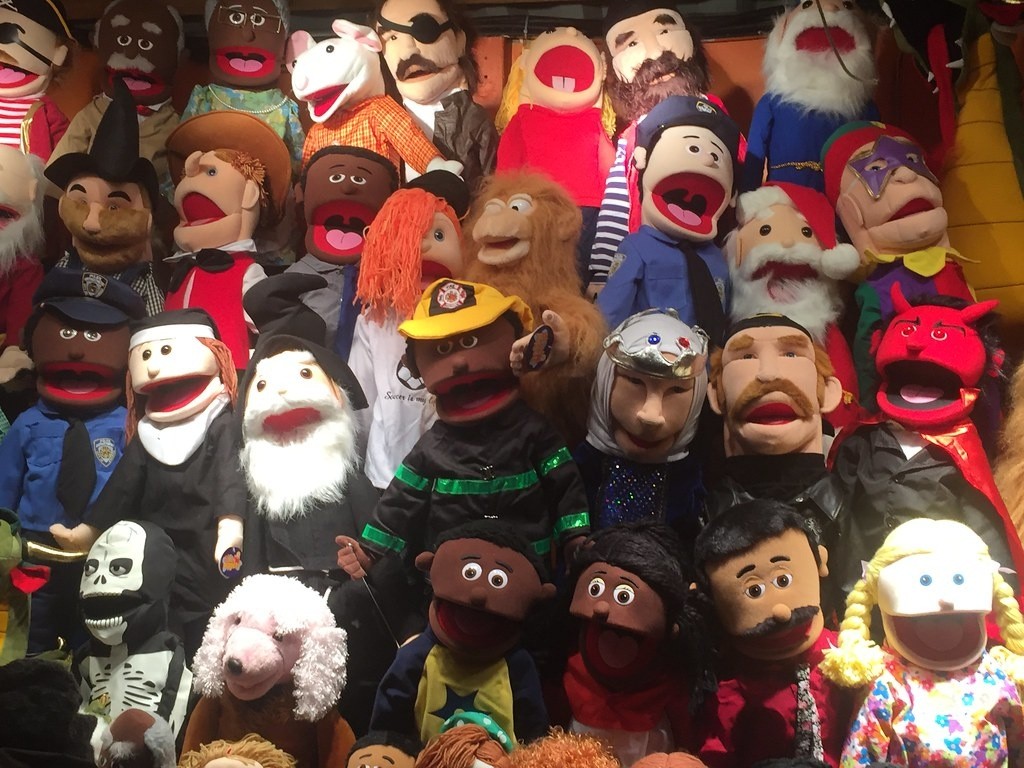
(1, 1), (881, 292)
(0, 73), (978, 489)
(1, 267), (1023, 768)
